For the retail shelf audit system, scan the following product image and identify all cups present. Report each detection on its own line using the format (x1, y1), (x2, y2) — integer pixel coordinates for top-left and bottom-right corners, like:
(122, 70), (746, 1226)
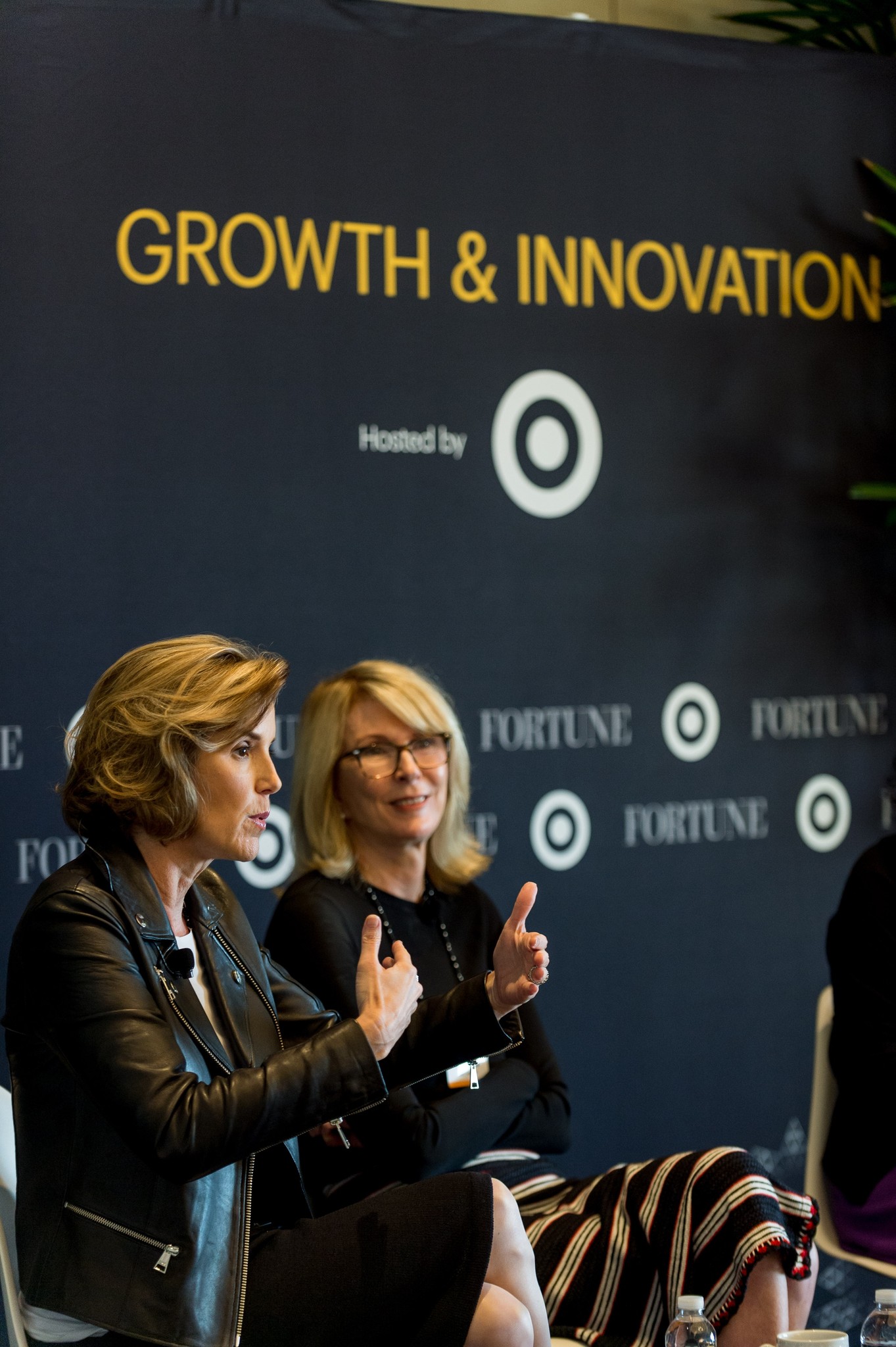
(759, 1330), (849, 1347)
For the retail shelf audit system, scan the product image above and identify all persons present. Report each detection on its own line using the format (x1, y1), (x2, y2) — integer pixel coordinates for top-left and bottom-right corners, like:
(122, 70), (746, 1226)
(824, 821), (896, 1265)
(5, 635), (550, 1347)
(265, 657), (822, 1347)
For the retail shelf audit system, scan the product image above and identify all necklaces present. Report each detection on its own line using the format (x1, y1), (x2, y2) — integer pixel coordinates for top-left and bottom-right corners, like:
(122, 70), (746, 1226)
(359, 877), (465, 1002)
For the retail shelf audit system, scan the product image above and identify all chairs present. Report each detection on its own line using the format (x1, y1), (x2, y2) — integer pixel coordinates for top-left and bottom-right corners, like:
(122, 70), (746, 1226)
(805, 989), (896, 1280)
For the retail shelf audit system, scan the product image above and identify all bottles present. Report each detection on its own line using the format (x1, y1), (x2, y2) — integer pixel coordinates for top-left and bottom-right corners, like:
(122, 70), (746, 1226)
(861, 1289), (896, 1347)
(665, 1296), (717, 1347)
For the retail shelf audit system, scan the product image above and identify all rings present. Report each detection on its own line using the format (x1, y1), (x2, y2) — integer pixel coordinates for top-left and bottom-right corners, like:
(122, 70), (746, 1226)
(527, 967), (549, 985)
(416, 975), (421, 984)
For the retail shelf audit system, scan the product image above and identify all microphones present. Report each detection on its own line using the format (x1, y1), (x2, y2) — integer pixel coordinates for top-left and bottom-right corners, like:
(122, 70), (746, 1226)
(172, 948), (195, 978)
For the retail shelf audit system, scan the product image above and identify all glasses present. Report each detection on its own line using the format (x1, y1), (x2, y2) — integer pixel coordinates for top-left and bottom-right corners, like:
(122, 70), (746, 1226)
(341, 734), (453, 780)
